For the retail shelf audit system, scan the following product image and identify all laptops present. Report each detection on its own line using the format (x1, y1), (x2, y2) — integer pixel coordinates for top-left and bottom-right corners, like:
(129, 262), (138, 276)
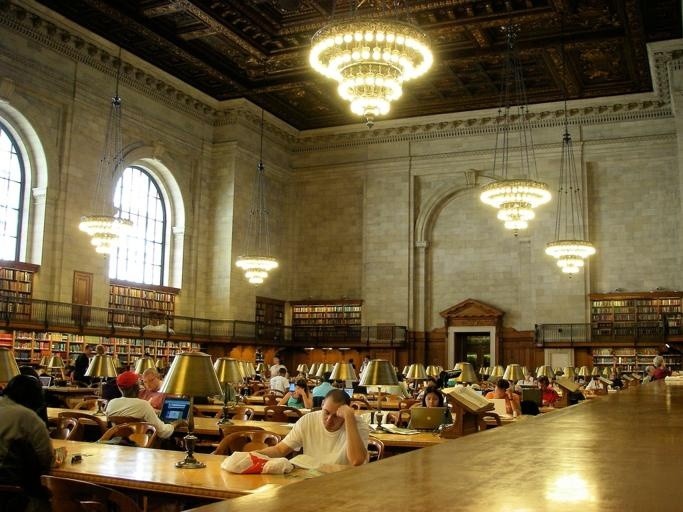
(344, 387), (354, 399)
(39, 377), (52, 388)
(411, 406), (447, 432)
(288, 382), (296, 391)
(159, 396), (191, 427)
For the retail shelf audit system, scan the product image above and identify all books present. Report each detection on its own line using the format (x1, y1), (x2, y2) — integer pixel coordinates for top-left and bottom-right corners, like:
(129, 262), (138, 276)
(368, 424), (370, 432)
(68, 332), (84, 343)
(13, 339), (32, 348)
(33, 342), (49, 350)
(180, 341), (192, 349)
(15, 271), (34, 282)
(156, 292), (175, 303)
(118, 355), (128, 362)
(258, 328), (283, 336)
(14, 330), (34, 339)
(103, 336), (115, 343)
(0, 301), (30, 315)
(591, 306), (683, 314)
(169, 341), (181, 348)
(129, 346), (142, 354)
(145, 347), (156, 355)
(293, 305), (361, 313)
(592, 321), (682, 329)
(110, 286), (130, 297)
(592, 299), (681, 307)
(34, 351), (50, 360)
(146, 339), (155, 345)
(141, 298), (174, 310)
(118, 304), (143, 312)
(35, 331), (50, 340)
(110, 295), (142, 306)
(73, 345), (83, 351)
(156, 340), (168, 347)
(289, 454), (343, 480)
(116, 338), (129, 344)
(131, 356), (141, 363)
(51, 343), (69, 352)
(295, 331), (360, 337)
(141, 308), (172, 316)
(169, 349), (181, 356)
(294, 319), (360, 324)
(15, 351), (30, 358)
(192, 343), (201, 350)
(294, 326), (360, 331)
(292, 313), (360, 319)
(0, 268), (14, 280)
(157, 349), (168, 356)
(50, 332), (68, 342)
(130, 337), (143, 345)
(126, 314), (142, 325)
(257, 303), (283, 310)
(1, 313), (29, 323)
(142, 290), (156, 299)
(407, 433), (414, 434)
(0, 290), (32, 304)
(69, 344), (73, 351)
(257, 312), (283, 319)
(257, 317), (283, 325)
(144, 316), (168, 326)
(110, 303), (118, 309)
(1, 280), (32, 293)
(407, 428), (420, 433)
(103, 345), (115, 353)
(108, 313), (126, 324)
(591, 312), (682, 321)
(116, 346), (129, 352)
(370, 424), (406, 434)
(129, 288), (141, 297)
(591, 328), (683, 336)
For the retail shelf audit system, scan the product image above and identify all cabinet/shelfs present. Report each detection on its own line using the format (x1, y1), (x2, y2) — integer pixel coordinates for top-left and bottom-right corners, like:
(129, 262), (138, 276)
(288, 297), (363, 342)
(0, 258), (40, 325)
(105, 279), (180, 330)
(587, 289), (682, 336)
(252, 294), (285, 341)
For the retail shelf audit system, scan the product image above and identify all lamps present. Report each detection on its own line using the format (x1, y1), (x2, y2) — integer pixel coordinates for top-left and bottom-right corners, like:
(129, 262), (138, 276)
(304, 1), (439, 134)
(539, 38), (596, 276)
(233, 59), (280, 289)
(475, 14), (550, 235)
(79, 43), (135, 258)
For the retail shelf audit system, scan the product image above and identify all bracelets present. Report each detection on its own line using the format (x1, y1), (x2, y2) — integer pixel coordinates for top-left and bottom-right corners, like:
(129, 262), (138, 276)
(508, 398), (513, 400)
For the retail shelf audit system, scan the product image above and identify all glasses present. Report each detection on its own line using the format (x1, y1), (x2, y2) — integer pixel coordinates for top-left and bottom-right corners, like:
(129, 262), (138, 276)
(72, 456), (81, 463)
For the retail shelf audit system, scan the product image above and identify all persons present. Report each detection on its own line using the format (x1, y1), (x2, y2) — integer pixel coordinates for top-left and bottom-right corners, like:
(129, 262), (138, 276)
(104, 370), (190, 449)
(347, 358), (356, 373)
(484, 378), (522, 416)
(136, 367), (164, 410)
(95, 345), (103, 356)
(357, 355), (370, 380)
(252, 388), (370, 466)
(536, 375), (560, 412)
(73, 344), (94, 385)
(407, 385), (454, 433)
(47, 351), (64, 368)
(533, 377), (538, 386)
(649, 356), (672, 380)
(0, 375), (66, 511)
(277, 378), (313, 410)
(268, 367), (290, 396)
(586, 375), (604, 390)
(516, 371), (534, 387)
(608, 371), (623, 391)
(311, 371), (339, 397)
(641, 365), (656, 384)
(269, 357), (288, 378)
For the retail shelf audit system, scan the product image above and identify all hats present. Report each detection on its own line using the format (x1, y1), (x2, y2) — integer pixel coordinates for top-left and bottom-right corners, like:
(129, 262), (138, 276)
(116, 371), (139, 385)
